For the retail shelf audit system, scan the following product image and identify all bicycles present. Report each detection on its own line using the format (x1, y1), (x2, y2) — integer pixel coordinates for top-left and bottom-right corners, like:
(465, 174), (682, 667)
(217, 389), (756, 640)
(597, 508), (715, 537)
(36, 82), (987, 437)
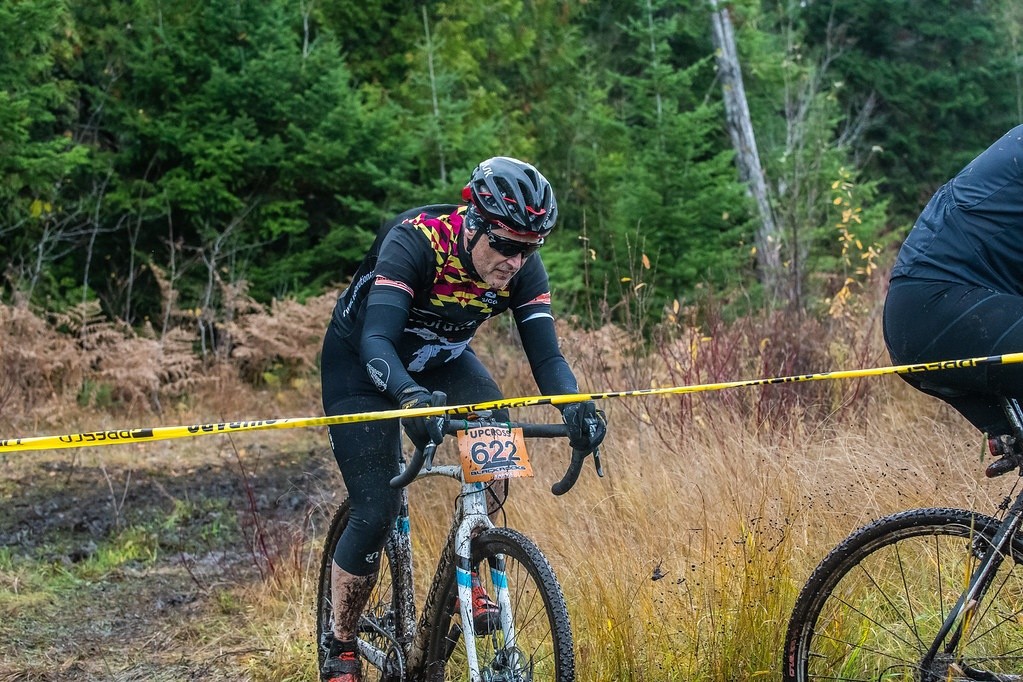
(783, 389), (1023, 682)
(314, 391), (606, 682)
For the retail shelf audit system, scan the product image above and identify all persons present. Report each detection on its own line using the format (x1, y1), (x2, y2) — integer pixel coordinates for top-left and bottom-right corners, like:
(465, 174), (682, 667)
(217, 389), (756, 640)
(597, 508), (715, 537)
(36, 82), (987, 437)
(883, 118), (1023, 474)
(322, 149), (606, 680)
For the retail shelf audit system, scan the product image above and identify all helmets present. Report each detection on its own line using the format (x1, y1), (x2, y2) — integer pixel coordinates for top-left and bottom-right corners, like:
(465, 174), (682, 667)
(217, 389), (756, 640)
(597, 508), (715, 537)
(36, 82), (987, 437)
(462, 157), (558, 238)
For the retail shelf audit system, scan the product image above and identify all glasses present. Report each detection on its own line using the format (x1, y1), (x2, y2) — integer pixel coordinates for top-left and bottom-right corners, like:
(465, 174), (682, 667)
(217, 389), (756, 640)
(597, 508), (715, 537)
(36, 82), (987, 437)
(484, 228), (544, 259)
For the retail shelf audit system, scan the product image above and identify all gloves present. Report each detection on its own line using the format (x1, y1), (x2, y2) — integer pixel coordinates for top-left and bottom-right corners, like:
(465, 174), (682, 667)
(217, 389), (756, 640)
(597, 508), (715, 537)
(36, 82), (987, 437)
(401, 391), (443, 444)
(562, 401), (607, 458)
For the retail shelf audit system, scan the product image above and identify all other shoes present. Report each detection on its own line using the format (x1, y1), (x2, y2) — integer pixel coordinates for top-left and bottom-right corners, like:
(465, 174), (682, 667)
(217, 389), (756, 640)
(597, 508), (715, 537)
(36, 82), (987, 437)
(453, 585), (502, 633)
(322, 638), (361, 682)
